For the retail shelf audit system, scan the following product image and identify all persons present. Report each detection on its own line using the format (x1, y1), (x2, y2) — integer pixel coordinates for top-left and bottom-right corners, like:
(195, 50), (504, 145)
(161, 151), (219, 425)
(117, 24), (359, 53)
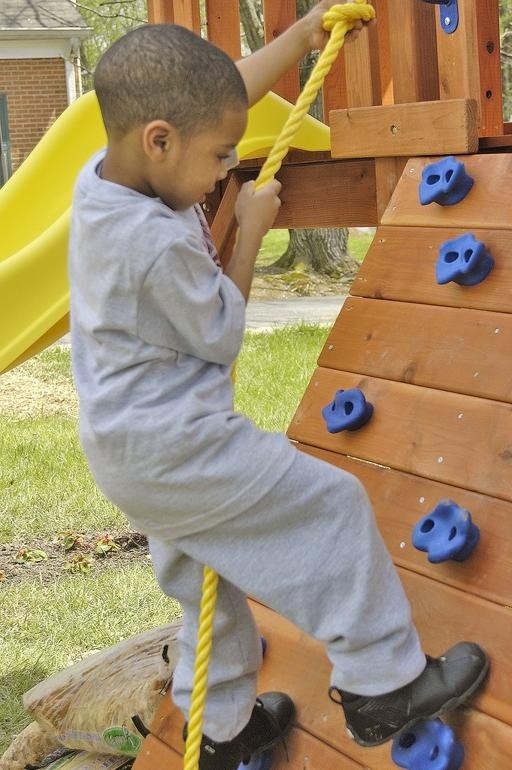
(69, 0), (489, 770)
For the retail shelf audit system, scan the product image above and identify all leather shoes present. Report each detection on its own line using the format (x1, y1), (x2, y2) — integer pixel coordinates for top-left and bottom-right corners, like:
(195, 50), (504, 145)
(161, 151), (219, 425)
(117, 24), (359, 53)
(181, 689), (298, 770)
(334, 638), (492, 748)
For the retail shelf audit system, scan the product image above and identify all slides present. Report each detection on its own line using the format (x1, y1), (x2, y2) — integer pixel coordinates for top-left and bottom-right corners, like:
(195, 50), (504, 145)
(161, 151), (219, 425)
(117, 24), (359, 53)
(0, 89), (331, 372)
